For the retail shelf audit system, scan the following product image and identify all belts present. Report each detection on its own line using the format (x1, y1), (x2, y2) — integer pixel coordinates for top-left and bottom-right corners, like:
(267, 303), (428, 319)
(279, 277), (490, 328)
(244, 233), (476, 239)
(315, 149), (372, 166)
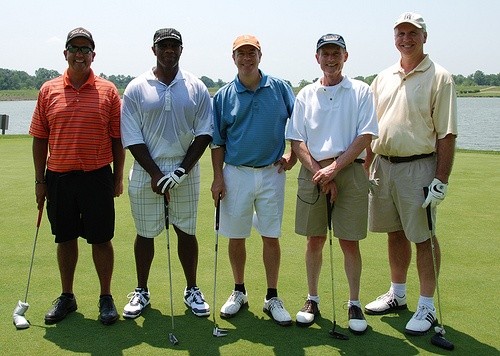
(241, 164), (269, 169)
(381, 152), (433, 163)
(329, 157), (365, 164)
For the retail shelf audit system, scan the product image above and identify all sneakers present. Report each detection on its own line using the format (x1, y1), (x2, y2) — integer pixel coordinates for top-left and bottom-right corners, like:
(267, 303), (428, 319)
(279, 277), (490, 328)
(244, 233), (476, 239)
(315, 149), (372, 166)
(220, 289), (248, 317)
(96, 294), (118, 325)
(404, 306), (438, 335)
(295, 299), (320, 325)
(343, 302), (367, 334)
(263, 297), (292, 326)
(123, 287), (150, 317)
(44, 294), (77, 321)
(364, 291), (407, 315)
(183, 285), (210, 316)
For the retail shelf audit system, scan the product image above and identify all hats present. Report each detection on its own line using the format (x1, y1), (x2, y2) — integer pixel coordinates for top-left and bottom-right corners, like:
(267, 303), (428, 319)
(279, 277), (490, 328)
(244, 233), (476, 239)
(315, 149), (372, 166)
(394, 11), (426, 32)
(66, 27), (94, 45)
(316, 34), (346, 51)
(153, 28), (183, 45)
(232, 34), (261, 51)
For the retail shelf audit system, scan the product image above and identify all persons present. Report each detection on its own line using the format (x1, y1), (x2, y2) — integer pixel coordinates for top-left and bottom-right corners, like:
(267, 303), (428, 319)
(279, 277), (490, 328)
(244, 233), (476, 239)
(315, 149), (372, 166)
(29, 27), (126, 325)
(364, 12), (458, 336)
(120, 28), (214, 319)
(209, 34), (297, 327)
(285, 34), (379, 336)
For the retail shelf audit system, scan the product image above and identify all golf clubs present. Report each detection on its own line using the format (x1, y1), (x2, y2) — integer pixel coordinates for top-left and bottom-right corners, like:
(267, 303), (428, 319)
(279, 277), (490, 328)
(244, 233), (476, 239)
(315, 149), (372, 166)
(24, 196), (43, 304)
(423, 186), (447, 335)
(325, 190), (350, 340)
(212, 194), (228, 336)
(164, 194), (179, 345)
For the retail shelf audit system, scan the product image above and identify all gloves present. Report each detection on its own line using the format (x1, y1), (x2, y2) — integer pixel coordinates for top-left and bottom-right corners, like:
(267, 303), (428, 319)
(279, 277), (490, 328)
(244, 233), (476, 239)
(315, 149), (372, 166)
(421, 177), (448, 209)
(157, 167), (189, 194)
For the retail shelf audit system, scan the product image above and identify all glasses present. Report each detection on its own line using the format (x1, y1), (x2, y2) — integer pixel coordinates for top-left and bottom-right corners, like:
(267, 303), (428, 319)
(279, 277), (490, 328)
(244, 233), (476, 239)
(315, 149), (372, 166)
(66, 45), (94, 54)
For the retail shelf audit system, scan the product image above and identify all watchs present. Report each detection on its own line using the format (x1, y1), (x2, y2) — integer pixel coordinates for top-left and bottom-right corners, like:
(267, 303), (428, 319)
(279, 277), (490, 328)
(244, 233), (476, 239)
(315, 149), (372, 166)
(35, 180), (46, 184)
